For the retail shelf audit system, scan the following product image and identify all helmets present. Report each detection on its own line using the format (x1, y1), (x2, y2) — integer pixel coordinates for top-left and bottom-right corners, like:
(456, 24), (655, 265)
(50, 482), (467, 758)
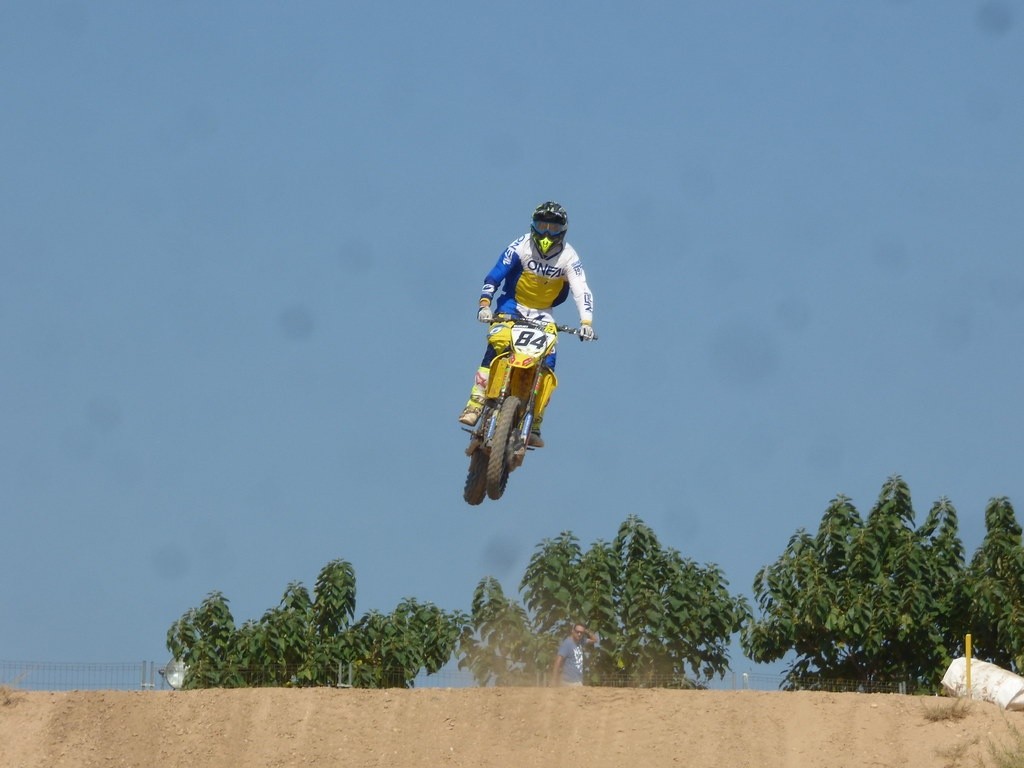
(531, 201), (568, 253)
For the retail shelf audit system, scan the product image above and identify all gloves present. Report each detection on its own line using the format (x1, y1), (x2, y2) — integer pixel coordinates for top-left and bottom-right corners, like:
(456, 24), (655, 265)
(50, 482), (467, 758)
(477, 306), (493, 323)
(578, 323), (594, 342)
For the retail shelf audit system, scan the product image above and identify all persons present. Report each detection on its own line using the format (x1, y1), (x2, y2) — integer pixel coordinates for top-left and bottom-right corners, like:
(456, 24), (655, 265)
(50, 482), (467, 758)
(459, 202), (596, 447)
(552, 623), (598, 688)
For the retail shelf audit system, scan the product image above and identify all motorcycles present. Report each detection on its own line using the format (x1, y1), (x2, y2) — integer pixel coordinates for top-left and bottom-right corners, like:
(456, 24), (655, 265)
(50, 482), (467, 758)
(460, 313), (598, 506)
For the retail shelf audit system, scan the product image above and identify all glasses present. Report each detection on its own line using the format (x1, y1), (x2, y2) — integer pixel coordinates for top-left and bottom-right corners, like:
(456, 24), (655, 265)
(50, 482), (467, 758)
(531, 223), (567, 236)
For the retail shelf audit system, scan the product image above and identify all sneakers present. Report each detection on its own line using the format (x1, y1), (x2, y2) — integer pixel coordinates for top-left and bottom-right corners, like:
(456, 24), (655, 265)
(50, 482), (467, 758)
(528, 430), (544, 447)
(458, 406), (482, 426)
